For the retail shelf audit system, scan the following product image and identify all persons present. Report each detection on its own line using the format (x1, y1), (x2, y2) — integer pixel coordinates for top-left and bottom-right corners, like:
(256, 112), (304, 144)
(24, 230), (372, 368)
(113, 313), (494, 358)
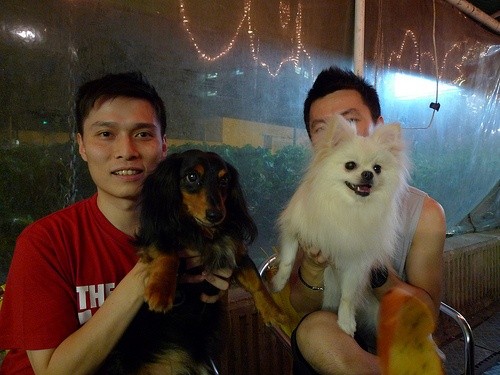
(0, 70), (233, 375)
(272, 67), (445, 375)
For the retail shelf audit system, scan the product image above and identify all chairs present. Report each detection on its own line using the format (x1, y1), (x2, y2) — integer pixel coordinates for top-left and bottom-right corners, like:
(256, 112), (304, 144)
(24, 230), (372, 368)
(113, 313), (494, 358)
(254, 254), (476, 375)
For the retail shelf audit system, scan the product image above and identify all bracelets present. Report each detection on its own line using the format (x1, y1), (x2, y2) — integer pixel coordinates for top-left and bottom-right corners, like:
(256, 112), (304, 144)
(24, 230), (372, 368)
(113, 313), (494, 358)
(298, 267), (324, 291)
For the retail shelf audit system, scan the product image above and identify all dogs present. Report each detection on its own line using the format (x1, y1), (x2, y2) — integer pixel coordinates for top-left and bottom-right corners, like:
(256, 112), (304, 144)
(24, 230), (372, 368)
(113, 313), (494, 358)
(272, 114), (411, 335)
(94, 147), (290, 375)
